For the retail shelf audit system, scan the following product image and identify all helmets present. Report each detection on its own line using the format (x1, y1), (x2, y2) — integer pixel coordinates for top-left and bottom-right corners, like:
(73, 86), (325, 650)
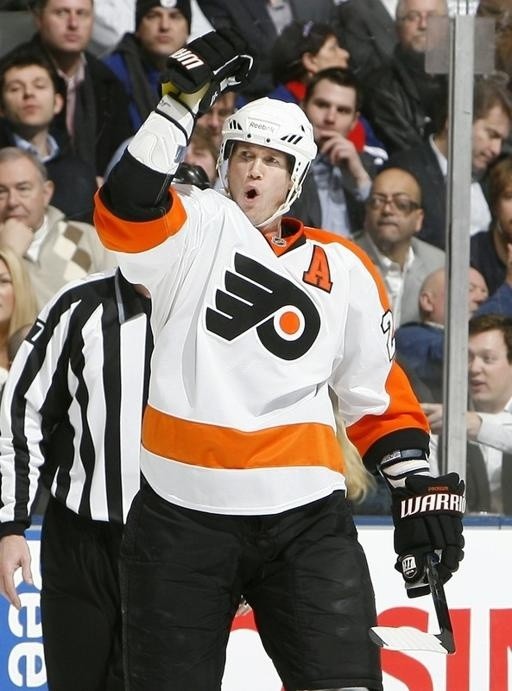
(217, 96), (320, 190)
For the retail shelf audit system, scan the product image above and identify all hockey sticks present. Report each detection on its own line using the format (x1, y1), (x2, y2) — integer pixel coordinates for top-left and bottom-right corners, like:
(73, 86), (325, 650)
(369, 551), (455, 656)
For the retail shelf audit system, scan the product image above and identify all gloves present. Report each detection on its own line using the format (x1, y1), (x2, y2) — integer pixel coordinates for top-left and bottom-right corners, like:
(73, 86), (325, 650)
(158, 25), (259, 117)
(392, 472), (467, 596)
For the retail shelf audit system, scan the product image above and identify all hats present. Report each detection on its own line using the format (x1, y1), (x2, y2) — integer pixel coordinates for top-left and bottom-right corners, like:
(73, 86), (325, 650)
(135, 0), (190, 32)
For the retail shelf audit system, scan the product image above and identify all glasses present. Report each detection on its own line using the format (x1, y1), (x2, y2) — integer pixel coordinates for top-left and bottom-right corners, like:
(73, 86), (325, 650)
(364, 194), (422, 213)
(401, 9), (440, 21)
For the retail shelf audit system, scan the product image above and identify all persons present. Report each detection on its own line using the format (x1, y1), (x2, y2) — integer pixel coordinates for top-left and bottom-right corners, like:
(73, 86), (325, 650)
(0, 164), (209, 689)
(0, 0), (512, 533)
(93, 29), (467, 689)
